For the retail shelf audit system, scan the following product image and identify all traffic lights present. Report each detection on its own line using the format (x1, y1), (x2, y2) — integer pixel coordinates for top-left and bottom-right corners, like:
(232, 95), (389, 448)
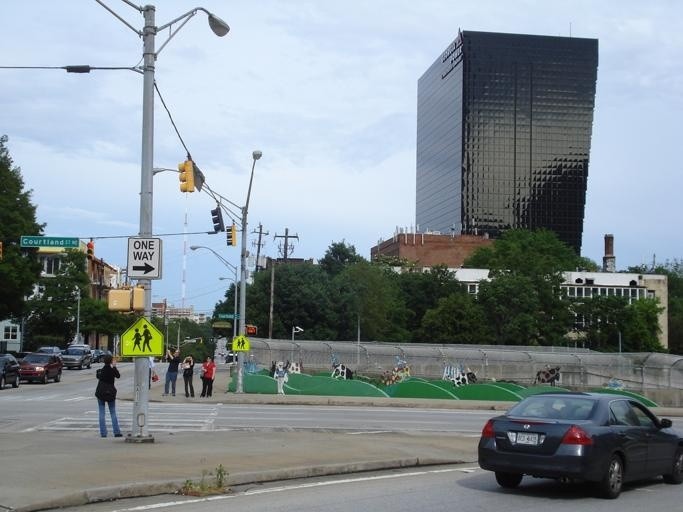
(178, 163), (187, 193)
(225, 227), (234, 248)
(246, 326), (256, 336)
(210, 207), (222, 233)
(86, 242), (92, 260)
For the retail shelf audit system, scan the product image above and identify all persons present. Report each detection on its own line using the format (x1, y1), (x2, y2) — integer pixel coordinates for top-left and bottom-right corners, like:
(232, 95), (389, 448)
(147, 355), (157, 391)
(198, 355), (216, 399)
(94, 355), (123, 437)
(179, 354), (195, 398)
(271, 359), (289, 395)
(161, 346), (180, 397)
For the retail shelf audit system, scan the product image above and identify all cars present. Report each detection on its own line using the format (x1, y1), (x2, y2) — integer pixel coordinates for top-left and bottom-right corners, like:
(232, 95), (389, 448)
(118, 341), (179, 363)
(477, 394), (683, 498)
(0, 344), (113, 390)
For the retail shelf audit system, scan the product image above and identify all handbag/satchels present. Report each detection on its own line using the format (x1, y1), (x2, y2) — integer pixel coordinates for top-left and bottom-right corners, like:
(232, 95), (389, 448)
(180, 362), (191, 370)
(151, 371), (159, 383)
(94, 379), (117, 402)
(198, 368), (208, 379)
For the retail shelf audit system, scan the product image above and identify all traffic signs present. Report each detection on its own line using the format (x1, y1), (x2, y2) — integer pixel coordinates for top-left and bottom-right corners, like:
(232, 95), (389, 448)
(20, 235), (79, 248)
(218, 313), (238, 319)
(127, 238), (160, 277)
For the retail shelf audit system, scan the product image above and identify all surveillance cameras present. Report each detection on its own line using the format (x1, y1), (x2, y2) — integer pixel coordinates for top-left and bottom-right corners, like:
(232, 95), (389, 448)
(296, 326), (304, 332)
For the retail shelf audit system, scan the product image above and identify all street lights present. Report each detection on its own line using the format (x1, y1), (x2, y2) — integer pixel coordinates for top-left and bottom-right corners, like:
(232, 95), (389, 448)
(136, 0), (230, 443)
(219, 277), (241, 290)
(190, 244), (238, 365)
(74, 284), (81, 334)
(153, 148), (260, 394)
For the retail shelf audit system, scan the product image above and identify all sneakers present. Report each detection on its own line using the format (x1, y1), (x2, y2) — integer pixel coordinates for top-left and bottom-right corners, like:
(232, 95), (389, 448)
(100, 434), (123, 438)
